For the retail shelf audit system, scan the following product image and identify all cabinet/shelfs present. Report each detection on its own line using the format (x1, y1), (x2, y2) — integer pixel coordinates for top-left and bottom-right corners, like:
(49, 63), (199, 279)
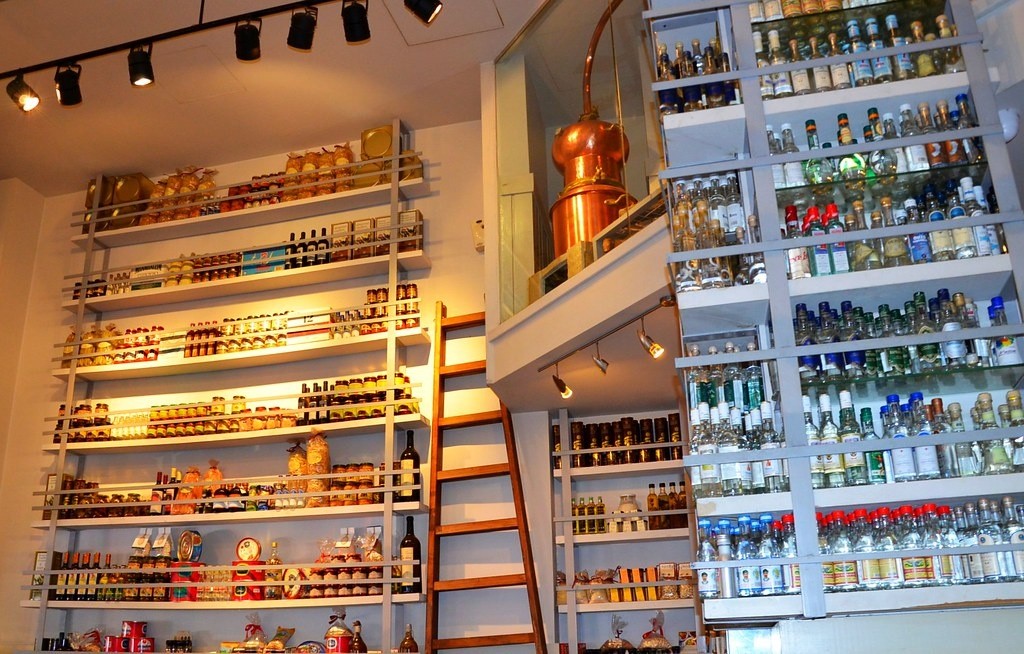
(641, 0), (1024, 616)
(17, 119), (434, 654)
(548, 403), (710, 654)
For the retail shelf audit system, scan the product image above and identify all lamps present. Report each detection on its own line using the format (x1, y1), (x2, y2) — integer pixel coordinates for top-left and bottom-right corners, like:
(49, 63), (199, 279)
(551, 363), (572, 398)
(590, 342), (610, 375)
(53, 63), (83, 108)
(234, 18), (263, 60)
(998, 107), (1021, 143)
(403, 0), (443, 24)
(126, 43), (155, 86)
(341, 0), (371, 42)
(6, 71), (40, 113)
(286, 5), (318, 51)
(635, 316), (665, 359)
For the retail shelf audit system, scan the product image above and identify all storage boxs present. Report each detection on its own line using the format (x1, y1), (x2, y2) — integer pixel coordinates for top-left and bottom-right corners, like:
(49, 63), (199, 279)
(240, 246), (285, 276)
(329, 221), (352, 262)
(374, 215), (391, 256)
(128, 263), (169, 292)
(398, 208), (423, 253)
(352, 218), (375, 260)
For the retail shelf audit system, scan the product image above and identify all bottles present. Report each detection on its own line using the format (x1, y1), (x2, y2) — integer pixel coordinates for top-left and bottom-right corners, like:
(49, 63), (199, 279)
(150, 466), (182, 516)
(296, 380), (333, 427)
(183, 319), (219, 358)
(549, 408), (688, 534)
(349, 620), (368, 653)
(638, 0), (1024, 593)
(56, 552), (125, 600)
(109, 412), (151, 439)
(285, 227), (330, 271)
(398, 624), (418, 654)
(400, 516), (422, 594)
(265, 542), (283, 600)
(332, 311), (361, 342)
(105, 272), (130, 296)
(400, 430), (418, 502)
(213, 483), (248, 509)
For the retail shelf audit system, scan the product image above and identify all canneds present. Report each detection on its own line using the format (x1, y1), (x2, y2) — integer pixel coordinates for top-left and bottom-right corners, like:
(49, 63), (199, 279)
(329, 372), (413, 423)
(308, 553), (383, 598)
(114, 325), (164, 363)
(122, 555), (170, 602)
(104, 620), (155, 652)
(219, 171), (287, 212)
(165, 260), (194, 286)
(59, 478), (150, 519)
(324, 635), (353, 654)
(329, 462), (375, 507)
(192, 253), (241, 283)
(216, 310), (294, 354)
(147, 395), (247, 439)
(72, 279), (107, 300)
(360, 283), (421, 335)
(53, 403), (111, 443)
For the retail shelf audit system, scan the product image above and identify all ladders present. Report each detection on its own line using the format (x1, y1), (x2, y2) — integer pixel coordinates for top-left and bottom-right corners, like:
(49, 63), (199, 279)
(424, 292), (548, 654)
